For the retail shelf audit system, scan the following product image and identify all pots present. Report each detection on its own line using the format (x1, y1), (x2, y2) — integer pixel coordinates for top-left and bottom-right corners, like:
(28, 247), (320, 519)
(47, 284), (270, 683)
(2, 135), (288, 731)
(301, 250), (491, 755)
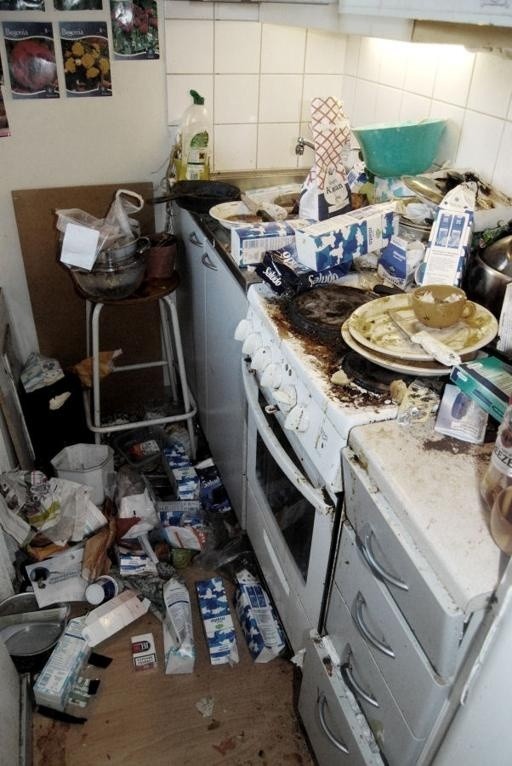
(147, 179), (242, 215)
(474, 230), (512, 306)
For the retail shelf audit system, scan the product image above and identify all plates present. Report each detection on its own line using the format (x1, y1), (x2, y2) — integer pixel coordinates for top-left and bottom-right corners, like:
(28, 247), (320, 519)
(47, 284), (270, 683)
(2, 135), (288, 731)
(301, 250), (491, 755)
(340, 314), (488, 379)
(349, 291), (497, 361)
(387, 173), (448, 243)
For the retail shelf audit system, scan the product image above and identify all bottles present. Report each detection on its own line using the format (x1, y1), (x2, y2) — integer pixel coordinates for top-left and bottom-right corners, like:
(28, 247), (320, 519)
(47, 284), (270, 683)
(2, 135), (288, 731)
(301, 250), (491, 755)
(479, 407), (512, 556)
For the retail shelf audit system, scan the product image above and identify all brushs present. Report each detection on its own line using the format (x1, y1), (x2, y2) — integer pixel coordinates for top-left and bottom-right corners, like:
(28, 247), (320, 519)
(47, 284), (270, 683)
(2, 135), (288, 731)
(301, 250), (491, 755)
(137, 534), (176, 581)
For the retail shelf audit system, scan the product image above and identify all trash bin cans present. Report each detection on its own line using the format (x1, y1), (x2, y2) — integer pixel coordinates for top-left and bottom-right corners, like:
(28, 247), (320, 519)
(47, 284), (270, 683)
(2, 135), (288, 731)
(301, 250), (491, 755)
(50, 443), (115, 506)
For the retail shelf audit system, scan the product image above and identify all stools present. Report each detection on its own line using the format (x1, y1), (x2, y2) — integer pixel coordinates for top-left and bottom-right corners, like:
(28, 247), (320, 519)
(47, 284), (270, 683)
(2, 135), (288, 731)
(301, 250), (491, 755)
(74, 269), (198, 463)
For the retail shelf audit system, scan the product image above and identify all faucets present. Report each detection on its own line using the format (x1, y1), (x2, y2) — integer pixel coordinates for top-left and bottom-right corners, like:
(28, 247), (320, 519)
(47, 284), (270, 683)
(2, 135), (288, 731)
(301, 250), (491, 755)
(295, 137), (316, 156)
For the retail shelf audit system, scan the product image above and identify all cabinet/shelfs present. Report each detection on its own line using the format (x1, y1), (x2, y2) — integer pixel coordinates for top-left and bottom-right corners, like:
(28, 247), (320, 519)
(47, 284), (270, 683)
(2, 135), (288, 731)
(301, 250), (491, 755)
(171, 205), (249, 529)
(297, 417), (512, 766)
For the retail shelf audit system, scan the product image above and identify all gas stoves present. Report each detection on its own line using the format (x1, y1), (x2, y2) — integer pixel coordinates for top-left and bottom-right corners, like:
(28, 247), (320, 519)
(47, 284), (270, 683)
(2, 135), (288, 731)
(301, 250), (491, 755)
(231, 271), (441, 495)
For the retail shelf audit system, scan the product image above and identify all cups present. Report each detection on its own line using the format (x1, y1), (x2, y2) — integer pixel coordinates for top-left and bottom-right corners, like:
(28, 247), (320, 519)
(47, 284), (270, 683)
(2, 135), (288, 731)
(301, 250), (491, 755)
(85, 575), (118, 607)
(144, 230), (179, 280)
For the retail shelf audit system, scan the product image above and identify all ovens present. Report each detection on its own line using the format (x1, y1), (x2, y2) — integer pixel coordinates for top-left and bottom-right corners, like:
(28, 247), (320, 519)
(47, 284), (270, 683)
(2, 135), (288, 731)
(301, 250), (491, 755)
(227, 354), (341, 664)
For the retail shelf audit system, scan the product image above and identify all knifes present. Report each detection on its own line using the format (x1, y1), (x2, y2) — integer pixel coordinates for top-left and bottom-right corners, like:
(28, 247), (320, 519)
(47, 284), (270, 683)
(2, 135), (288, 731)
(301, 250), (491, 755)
(386, 308), (461, 368)
(237, 193), (275, 224)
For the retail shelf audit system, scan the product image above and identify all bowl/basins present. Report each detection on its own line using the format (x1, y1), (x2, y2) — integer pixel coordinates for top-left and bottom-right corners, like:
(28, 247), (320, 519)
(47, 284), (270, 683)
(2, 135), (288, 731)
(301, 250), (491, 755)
(1, 591), (69, 660)
(210, 200), (288, 230)
(351, 117), (446, 178)
(63, 230), (151, 269)
(70, 256), (147, 301)
